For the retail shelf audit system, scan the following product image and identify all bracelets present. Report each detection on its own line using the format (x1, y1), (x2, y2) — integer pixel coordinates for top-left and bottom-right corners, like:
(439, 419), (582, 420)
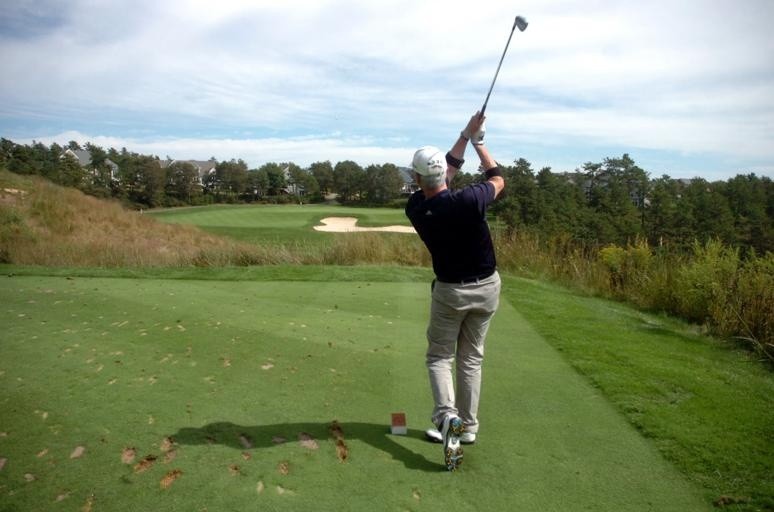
(445, 152), (466, 168)
(484, 166), (503, 179)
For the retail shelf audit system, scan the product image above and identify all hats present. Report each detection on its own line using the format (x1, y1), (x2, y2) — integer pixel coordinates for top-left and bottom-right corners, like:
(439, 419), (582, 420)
(407, 144), (449, 178)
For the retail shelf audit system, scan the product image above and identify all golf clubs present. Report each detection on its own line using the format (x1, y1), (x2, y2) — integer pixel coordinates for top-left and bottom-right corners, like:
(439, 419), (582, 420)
(480, 16), (528, 119)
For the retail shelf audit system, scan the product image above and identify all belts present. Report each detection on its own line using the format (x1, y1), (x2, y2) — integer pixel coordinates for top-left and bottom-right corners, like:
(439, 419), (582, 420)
(436, 268), (496, 286)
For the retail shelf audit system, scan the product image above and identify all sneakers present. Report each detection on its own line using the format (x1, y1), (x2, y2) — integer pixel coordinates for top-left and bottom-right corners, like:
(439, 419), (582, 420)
(423, 425), (477, 446)
(439, 411), (465, 474)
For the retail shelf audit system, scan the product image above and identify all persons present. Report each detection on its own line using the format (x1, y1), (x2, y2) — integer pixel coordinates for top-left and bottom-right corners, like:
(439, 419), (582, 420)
(402, 108), (508, 472)
